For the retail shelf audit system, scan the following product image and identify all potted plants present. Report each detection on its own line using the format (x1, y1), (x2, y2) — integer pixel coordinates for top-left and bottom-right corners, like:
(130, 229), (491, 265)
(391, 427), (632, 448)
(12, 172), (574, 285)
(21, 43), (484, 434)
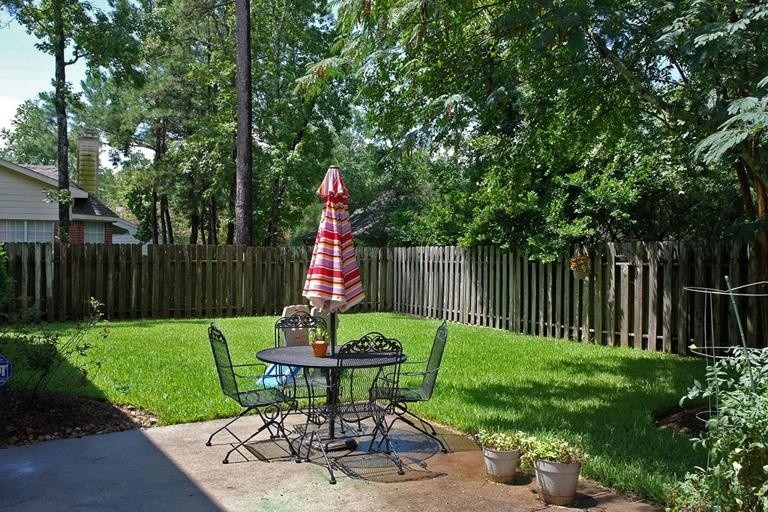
(474, 425), (591, 505)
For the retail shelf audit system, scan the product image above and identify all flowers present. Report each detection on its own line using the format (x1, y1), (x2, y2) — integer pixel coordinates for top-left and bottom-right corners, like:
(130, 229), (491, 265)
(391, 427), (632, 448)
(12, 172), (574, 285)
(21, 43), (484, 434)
(567, 254), (592, 276)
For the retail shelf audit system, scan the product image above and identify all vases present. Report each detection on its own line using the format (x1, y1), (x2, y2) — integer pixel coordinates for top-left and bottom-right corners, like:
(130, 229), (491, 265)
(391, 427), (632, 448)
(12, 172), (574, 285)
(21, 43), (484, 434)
(573, 268), (585, 281)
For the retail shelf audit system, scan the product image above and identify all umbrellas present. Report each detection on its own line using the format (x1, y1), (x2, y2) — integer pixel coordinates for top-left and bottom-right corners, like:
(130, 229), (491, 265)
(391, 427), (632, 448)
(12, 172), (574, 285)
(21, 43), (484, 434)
(301, 163), (369, 438)
